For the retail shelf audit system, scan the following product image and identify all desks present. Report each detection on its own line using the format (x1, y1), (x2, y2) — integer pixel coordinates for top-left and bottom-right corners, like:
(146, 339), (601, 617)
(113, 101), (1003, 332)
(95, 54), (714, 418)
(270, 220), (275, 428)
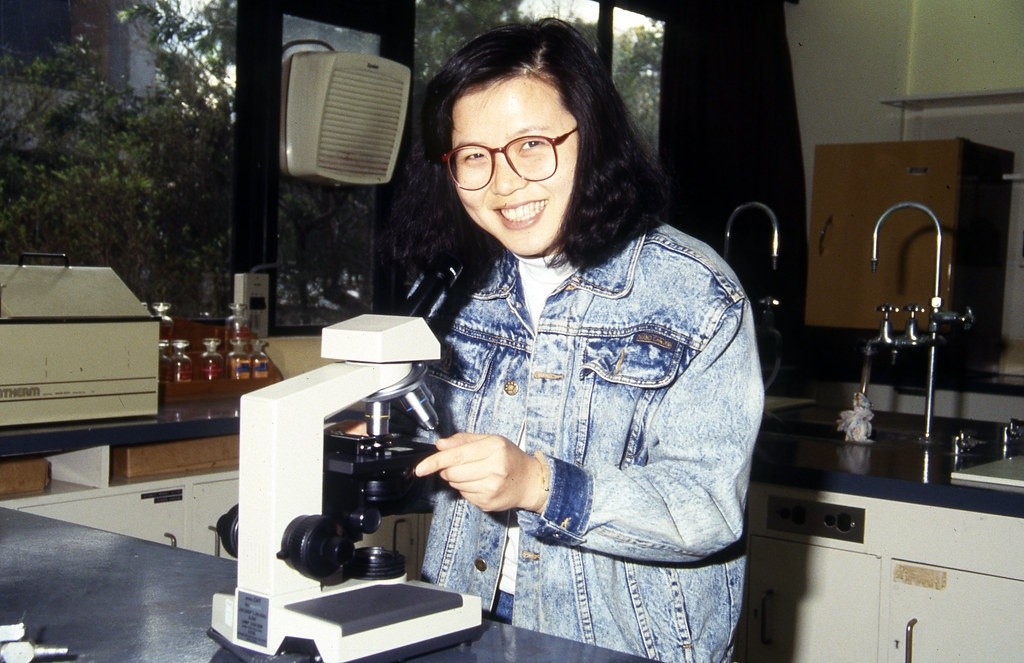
(0, 501), (666, 663)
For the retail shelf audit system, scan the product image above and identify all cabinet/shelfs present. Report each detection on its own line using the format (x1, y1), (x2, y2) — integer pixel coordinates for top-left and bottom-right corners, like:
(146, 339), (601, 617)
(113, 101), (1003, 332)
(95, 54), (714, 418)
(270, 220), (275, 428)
(0, 471), (240, 559)
(803, 136), (1015, 342)
(734, 473), (1024, 663)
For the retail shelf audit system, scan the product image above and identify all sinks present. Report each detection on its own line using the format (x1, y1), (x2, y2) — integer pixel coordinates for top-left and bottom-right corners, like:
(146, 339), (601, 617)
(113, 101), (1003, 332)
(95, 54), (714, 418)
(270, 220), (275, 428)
(757, 416), (908, 445)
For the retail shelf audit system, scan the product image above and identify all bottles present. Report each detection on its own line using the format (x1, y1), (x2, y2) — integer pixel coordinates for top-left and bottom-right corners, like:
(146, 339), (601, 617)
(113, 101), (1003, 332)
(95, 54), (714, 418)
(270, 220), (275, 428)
(152, 302), (270, 382)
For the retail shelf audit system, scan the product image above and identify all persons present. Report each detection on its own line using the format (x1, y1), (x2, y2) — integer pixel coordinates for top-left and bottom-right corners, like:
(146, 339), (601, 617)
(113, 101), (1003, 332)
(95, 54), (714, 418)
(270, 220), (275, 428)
(323, 16), (766, 662)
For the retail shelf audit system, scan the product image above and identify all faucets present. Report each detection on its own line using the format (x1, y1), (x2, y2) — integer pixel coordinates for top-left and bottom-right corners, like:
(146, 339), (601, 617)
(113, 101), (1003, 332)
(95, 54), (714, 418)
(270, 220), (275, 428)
(862, 302), (904, 358)
(871, 202), (942, 276)
(753, 293), (784, 349)
(891, 304), (926, 366)
(722, 201), (779, 269)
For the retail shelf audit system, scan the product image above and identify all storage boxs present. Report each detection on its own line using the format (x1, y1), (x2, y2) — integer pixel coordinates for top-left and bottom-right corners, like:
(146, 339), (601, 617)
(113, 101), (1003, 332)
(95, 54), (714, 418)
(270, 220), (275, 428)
(0, 454), (52, 497)
(110, 432), (240, 478)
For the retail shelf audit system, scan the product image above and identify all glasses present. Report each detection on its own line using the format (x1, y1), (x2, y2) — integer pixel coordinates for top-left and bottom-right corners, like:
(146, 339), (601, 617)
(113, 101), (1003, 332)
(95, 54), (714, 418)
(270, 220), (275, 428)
(441, 128), (578, 190)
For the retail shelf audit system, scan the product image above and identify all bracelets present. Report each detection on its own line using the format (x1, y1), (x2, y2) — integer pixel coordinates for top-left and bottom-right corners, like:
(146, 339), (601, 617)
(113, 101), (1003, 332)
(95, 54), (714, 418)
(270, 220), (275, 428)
(527, 449), (551, 513)
